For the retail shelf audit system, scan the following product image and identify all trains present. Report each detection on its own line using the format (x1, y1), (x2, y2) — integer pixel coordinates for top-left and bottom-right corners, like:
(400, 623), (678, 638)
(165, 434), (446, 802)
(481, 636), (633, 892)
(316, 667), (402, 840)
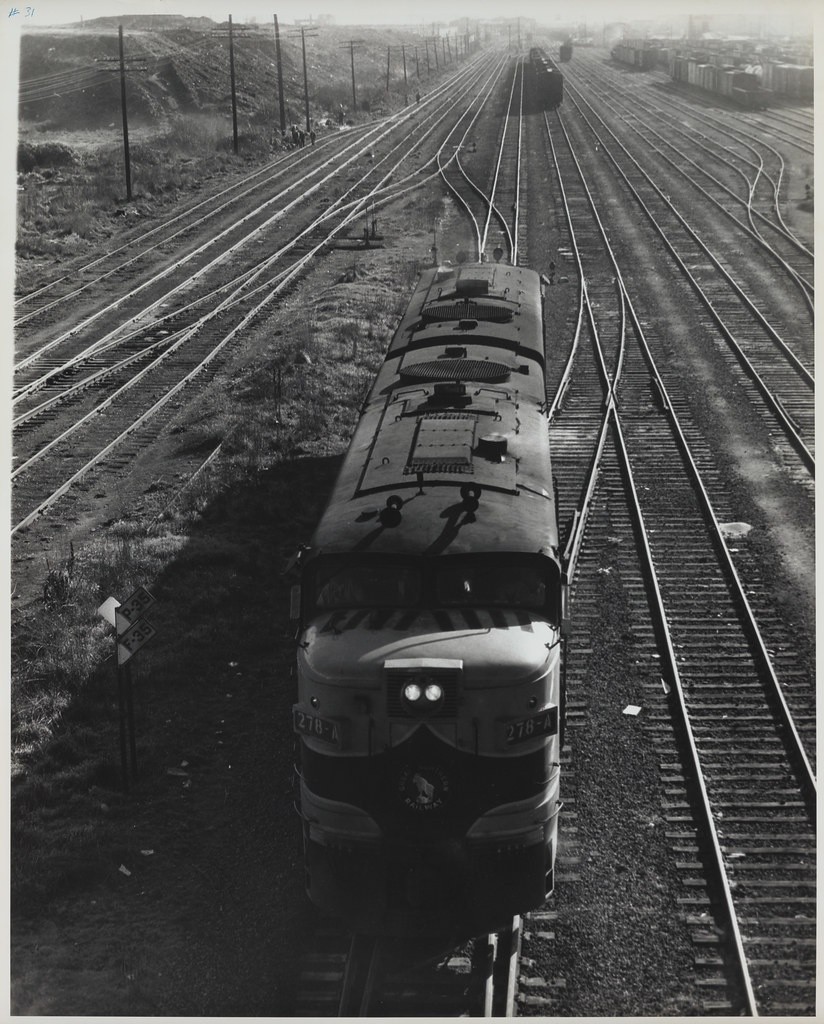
(288, 252), (569, 915)
(529, 46), (564, 107)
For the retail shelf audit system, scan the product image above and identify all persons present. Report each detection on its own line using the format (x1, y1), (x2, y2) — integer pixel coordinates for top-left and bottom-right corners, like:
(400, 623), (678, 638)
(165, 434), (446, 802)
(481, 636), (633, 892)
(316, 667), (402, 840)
(274, 91), (420, 148)
